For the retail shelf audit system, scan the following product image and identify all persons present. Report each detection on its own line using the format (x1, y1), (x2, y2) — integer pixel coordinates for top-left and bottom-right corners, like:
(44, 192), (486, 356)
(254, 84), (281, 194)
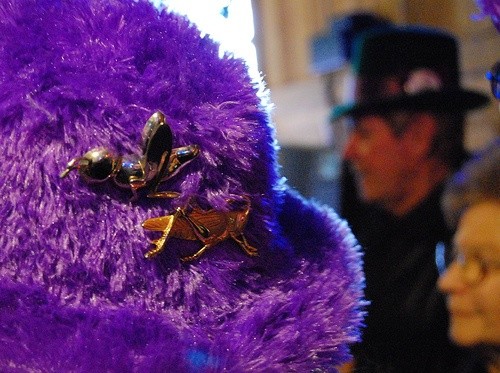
(329, 25), (500, 373)
(438, 137), (500, 347)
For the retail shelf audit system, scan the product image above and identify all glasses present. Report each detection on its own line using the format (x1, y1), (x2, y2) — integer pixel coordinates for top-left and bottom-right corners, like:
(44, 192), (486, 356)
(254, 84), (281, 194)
(434, 242), (500, 288)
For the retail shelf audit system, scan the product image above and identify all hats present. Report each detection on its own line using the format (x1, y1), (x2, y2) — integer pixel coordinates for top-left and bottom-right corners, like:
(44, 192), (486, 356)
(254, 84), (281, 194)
(325, 24), (491, 127)
(0, 1), (368, 373)
